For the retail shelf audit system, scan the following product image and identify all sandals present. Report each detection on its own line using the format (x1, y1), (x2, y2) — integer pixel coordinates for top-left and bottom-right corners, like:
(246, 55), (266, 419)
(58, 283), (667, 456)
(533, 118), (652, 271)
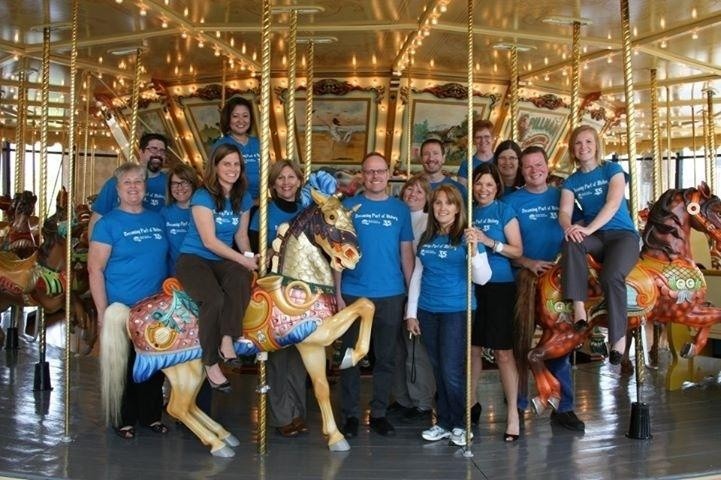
(139, 418), (168, 433)
(112, 422), (136, 439)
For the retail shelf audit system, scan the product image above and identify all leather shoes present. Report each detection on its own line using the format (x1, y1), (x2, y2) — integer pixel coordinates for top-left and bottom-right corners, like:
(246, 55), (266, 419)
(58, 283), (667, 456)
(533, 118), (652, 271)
(574, 309), (589, 331)
(609, 347), (623, 365)
(202, 365), (233, 394)
(217, 344), (243, 371)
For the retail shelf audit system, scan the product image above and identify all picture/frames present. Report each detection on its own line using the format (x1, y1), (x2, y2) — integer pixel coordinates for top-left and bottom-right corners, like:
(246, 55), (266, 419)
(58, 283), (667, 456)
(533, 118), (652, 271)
(392, 83), (502, 178)
(274, 79), (386, 172)
(112, 94), (181, 166)
(495, 93), (570, 163)
(176, 83), (260, 165)
(552, 107), (611, 176)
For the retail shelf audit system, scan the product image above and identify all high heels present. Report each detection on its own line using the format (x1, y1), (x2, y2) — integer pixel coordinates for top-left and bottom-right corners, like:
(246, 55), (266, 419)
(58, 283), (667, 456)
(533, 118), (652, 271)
(470, 401), (483, 426)
(502, 433), (520, 442)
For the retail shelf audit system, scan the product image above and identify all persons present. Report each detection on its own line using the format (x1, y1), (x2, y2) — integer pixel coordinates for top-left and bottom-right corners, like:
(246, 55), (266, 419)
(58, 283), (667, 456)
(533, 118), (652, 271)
(421, 138), (468, 200)
(457, 120), (496, 188)
(248, 158), (310, 436)
(174, 143), (261, 393)
(388, 176), (435, 421)
(501, 146), (585, 433)
(471, 163), (523, 442)
(213, 96), (260, 200)
(403, 184), (492, 448)
(492, 139), (522, 195)
(86, 163), (167, 439)
(158, 165), (212, 416)
(88, 133), (170, 245)
(558, 125), (639, 364)
(332, 153), (415, 439)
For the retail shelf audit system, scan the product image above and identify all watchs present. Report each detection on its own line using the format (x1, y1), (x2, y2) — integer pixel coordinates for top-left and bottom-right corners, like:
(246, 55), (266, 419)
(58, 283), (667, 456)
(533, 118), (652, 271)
(494, 240), (504, 253)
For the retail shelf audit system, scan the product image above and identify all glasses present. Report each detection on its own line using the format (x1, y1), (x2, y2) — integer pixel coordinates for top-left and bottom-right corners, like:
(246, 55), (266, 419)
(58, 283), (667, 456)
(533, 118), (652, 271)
(364, 169), (387, 175)
(144, 145), (165, 154)
(498, 155), (518, 161)
(170, 180), (190, 187)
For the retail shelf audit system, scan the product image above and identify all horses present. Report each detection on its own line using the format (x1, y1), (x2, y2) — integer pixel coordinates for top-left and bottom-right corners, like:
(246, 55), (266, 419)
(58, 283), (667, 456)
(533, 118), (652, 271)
(99, 187), (376, 459)
(515, 182), (717, 417)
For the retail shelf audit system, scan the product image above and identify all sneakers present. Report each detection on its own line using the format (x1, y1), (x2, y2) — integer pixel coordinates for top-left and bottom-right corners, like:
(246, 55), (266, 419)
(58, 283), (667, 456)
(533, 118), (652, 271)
(421, 425), (451, 441)
(402, 408), (433, 425)
(275, 423), (298, 438)
(550, 408), (585, 431)
(448, 428), (474, 447)
(337, 417), (359, 438)
(386, 400), (410, 415)
(292, 417), (311, 433)
(368, 415), (396, 436)
